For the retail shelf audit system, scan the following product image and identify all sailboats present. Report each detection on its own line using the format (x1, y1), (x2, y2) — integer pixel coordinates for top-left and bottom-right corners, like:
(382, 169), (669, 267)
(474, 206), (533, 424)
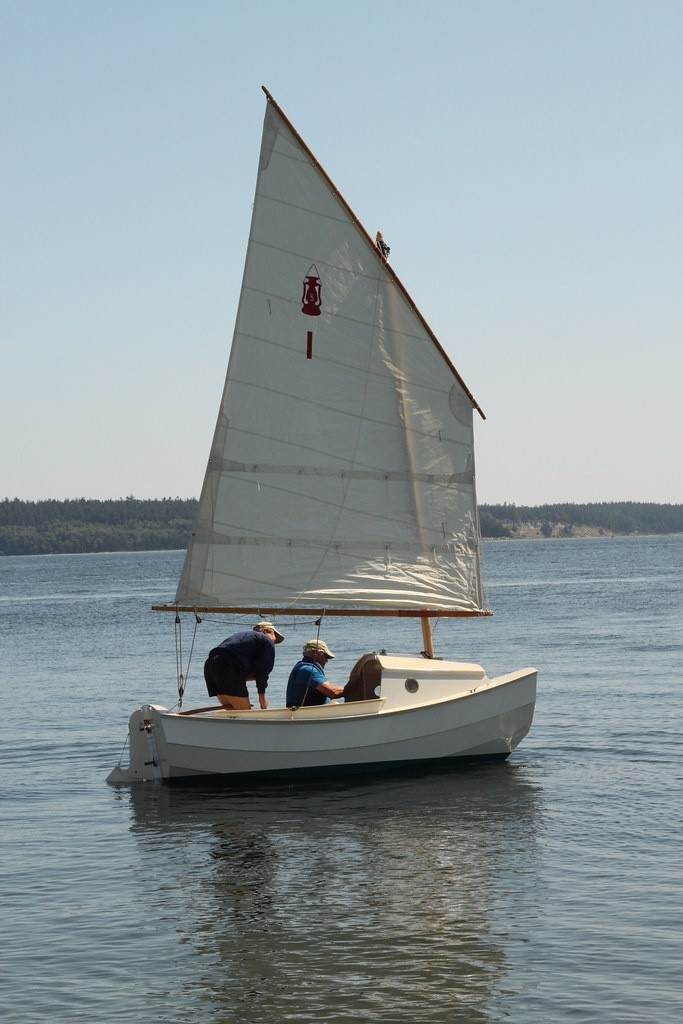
(106, 84), (537, 789)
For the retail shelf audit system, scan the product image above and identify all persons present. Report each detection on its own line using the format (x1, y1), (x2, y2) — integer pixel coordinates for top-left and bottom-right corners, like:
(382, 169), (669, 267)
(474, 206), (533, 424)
(285, 639), (345, 710)
(202, 621), (285, 711)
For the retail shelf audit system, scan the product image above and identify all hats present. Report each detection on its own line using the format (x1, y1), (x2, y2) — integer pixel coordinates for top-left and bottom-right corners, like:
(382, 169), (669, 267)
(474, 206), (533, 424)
(253, 622), (284, 644)
(304, 640), (334, 659)
